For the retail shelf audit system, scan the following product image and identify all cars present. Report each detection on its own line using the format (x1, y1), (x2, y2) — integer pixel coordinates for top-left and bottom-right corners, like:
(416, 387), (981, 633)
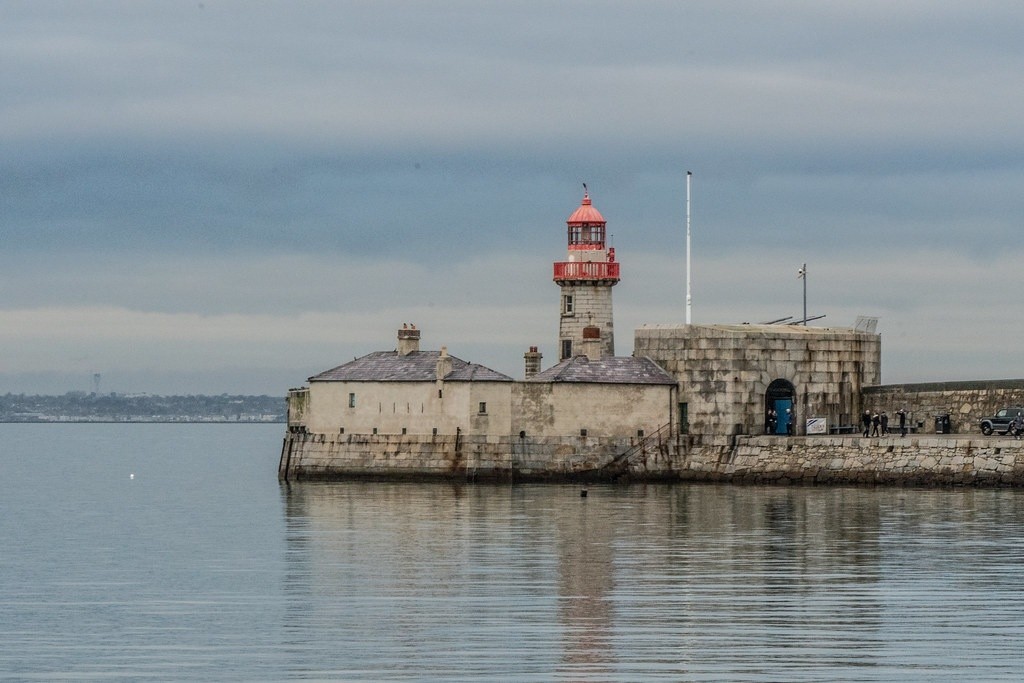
(980, 408), (1024, 436)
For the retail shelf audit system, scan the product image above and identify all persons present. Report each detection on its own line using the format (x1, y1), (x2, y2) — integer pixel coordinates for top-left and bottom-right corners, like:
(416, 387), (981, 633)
(1013, 411), (1024, 439)
(870, 412), (880, 437)
(895, 409), (907, 437)
(784, 409), (793, 437)
(765, 409), (778, 433)
(863, 410), (871, 438)
(880, 412), (889, 437)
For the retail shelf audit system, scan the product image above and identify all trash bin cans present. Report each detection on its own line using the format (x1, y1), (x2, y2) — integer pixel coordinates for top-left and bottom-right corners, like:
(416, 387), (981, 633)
(935, 414), (950, 434)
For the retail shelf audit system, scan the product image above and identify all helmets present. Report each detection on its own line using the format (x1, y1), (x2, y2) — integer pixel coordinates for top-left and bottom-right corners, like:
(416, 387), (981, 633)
(786, 409), (791, 414)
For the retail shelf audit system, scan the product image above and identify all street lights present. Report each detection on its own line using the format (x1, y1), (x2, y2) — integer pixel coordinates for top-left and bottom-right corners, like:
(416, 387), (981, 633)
(799, 263), (807, 327)
